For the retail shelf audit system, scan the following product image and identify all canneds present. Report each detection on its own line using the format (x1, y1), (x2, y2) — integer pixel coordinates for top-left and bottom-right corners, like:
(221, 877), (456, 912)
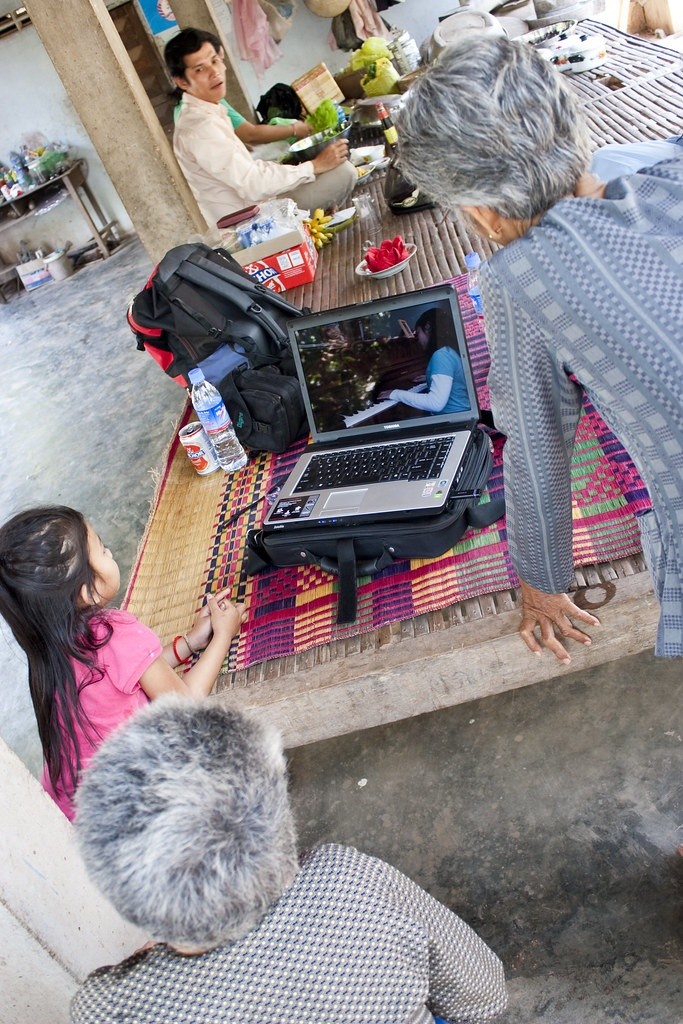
(178, 421), (221, 476)
(1, 184), (13, 200)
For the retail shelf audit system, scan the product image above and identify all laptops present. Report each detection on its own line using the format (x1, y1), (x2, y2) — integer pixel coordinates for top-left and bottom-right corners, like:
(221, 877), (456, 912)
(264, 281), (480, 532)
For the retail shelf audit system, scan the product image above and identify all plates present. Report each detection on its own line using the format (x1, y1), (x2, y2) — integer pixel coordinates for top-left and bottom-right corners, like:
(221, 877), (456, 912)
(355, 164), (375, 182)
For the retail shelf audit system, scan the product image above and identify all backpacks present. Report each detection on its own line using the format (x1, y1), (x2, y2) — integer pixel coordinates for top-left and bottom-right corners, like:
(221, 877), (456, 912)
(125, 242), (311, 397)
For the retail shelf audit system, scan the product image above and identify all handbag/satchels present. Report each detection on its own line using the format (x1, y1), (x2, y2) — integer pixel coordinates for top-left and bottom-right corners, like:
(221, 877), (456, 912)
(216, 363), (307, 453)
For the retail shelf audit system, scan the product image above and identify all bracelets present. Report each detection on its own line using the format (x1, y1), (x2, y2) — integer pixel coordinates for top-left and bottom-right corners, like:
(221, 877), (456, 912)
(173, 635), (189, 663)
(183, 634), (195, 653)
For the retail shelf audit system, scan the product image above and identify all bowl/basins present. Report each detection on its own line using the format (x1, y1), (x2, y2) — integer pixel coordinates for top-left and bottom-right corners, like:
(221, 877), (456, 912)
(369, 157), (390, 170)
(287, 121), (353, 161)
(354, 243), (418, 279)
(511, 19), (578, 49)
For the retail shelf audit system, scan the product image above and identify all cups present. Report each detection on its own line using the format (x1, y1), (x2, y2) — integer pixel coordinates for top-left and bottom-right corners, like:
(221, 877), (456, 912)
(351, 192), (382, 236)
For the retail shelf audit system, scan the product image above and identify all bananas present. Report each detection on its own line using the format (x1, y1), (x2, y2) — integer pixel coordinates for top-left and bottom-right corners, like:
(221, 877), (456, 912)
(302, 207), (335, 249)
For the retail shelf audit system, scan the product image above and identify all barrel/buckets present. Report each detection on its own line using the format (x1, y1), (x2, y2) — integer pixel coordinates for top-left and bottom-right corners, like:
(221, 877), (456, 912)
(42, 251), (74, 282)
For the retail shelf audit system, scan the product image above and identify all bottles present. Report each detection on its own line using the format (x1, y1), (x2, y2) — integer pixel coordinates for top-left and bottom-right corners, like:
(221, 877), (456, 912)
(333, 100), (348, 123)
(188, 368), (248, 473)
(465, 252), (494, 354)
(0, 145), (51, 199)
(375, 102), (399, 149)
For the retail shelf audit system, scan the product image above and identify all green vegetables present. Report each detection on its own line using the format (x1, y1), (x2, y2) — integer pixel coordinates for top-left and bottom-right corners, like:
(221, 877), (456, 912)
(363, 64), (376, 86)
(306, 95), (351, 142)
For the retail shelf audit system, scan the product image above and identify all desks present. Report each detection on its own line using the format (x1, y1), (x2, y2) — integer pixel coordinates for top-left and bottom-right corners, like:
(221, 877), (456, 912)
(0, 159), (118, 259)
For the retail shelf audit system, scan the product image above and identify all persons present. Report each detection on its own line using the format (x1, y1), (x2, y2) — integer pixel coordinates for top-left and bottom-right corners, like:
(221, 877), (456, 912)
(163, 26), (358, 242)
(327, 323), (348, 348)
(395, 35), (682, 665)
(376, 308), (471, 416)
(0, 504), (247, 822)
(68, 692), (509, 1024)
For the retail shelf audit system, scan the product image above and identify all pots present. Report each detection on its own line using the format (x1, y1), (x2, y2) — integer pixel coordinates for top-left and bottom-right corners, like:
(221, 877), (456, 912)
(542, 33), (608, 73)
